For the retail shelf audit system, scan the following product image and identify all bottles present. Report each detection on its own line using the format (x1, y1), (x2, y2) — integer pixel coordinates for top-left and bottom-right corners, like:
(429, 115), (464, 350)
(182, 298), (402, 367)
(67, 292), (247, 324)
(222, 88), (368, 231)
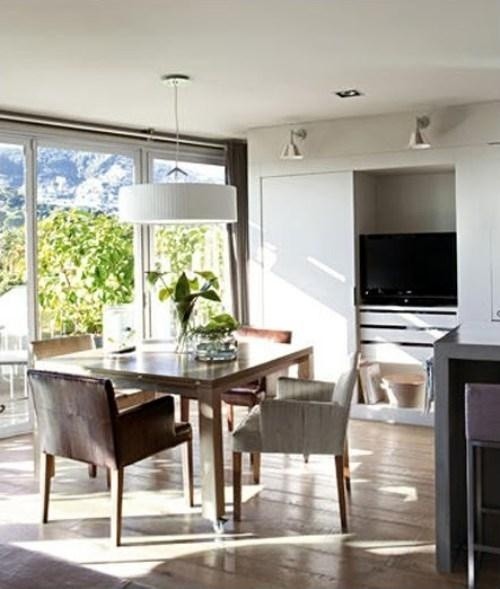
(102, 306), (133, 353)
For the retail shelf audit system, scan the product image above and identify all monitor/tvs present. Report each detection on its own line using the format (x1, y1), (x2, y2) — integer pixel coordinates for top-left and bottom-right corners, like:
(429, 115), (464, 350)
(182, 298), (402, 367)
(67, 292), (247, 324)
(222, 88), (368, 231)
(358, 232), (457, 307)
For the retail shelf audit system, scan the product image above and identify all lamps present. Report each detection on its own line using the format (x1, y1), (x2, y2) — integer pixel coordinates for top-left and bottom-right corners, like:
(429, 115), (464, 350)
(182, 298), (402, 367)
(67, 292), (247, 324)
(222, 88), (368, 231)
(118, 73), (238, 225)
(408, 113), (431, 149)
(281, 127), (306, 160)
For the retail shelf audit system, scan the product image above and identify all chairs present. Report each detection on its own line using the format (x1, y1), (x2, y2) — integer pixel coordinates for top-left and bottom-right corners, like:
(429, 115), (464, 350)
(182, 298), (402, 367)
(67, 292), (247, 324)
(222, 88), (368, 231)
(464, 383), (500, 589)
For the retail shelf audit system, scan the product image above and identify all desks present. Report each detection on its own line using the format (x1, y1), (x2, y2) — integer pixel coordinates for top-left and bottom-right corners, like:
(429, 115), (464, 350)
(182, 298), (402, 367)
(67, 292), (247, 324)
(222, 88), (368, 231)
(434, 322), (500, 574)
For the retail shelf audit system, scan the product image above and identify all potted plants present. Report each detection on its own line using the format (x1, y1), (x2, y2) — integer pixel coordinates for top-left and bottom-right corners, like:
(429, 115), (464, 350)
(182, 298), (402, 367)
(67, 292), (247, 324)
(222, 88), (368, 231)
(145, 266), (240, 360)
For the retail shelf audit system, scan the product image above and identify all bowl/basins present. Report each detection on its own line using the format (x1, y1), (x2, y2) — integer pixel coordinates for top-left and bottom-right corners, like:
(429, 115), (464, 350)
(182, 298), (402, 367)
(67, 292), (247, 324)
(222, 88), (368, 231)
(381, 372), (425, 407)
